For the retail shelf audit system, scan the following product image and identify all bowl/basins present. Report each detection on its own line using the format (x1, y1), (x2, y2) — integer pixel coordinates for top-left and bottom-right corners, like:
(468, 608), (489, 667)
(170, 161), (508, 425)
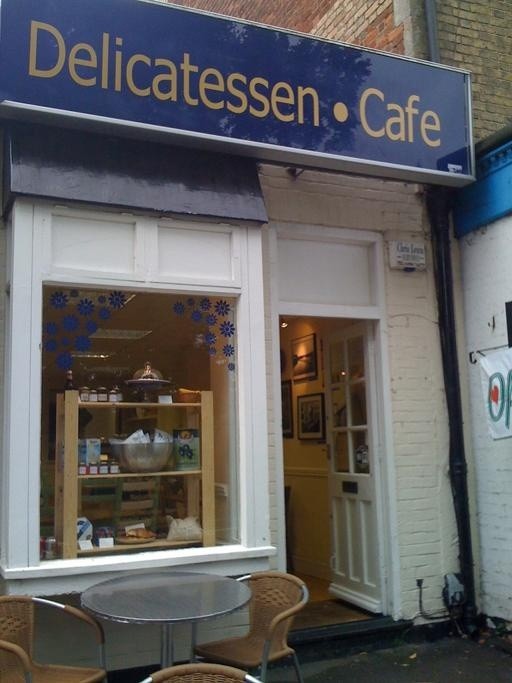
(113, 442), (173, 474)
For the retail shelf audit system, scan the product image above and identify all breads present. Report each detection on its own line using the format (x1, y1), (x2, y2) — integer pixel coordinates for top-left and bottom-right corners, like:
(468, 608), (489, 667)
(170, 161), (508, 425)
(114, 527), (159, 541)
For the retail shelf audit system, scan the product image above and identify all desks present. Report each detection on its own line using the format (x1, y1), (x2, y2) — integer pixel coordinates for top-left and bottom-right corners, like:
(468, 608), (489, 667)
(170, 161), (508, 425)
(80, 571), (255, 670)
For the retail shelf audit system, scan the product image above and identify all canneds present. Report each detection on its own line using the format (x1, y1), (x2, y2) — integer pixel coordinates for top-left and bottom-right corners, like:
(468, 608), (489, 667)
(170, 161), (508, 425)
(79, 462), (87, 475)
(79, 386), (123, 402)
(45, 537), (56, 560)
(99, 462), (108, 474)
(89, 461), (97, 474)
(40, 536), (45, 559)
(110, 462), (120, 473)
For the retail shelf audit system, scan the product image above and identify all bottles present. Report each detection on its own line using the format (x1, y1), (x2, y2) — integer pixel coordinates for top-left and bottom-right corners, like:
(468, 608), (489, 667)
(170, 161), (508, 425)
(66, 370), (73, 389)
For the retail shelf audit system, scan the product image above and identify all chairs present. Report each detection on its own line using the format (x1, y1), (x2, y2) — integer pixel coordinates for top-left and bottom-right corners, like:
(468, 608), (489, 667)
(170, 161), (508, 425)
(140, 661), (264, 683)
(1, 593), (111, 682)
(191, 570), (313, 683)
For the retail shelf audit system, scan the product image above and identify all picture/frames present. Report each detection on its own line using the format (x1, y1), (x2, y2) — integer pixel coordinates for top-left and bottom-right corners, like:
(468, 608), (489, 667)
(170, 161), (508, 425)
(282, 333), (324, 440)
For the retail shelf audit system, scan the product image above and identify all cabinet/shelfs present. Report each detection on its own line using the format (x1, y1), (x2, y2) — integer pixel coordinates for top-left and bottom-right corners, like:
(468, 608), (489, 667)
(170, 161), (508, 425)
(53, 389), (216, 558)
